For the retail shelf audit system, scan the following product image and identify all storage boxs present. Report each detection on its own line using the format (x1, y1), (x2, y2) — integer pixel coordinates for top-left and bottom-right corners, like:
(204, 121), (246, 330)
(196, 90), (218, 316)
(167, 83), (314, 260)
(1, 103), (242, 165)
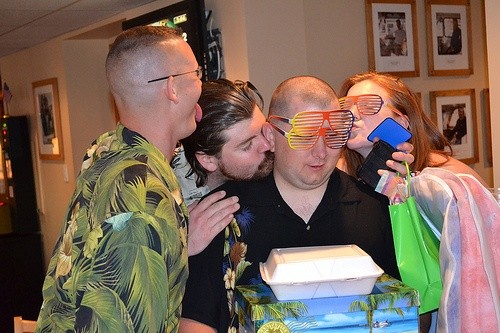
(234, 273), (420, 333)
(259, 244), (384, 301)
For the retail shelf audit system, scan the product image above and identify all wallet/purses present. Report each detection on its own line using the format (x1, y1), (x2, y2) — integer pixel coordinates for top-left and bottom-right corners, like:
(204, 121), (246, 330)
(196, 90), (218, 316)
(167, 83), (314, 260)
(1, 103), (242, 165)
(356, 140), (406, 189)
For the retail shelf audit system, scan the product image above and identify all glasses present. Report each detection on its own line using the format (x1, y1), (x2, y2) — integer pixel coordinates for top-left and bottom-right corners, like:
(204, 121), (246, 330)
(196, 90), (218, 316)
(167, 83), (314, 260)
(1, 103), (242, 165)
(266, 107), (354, 152)
(337, 94), (411, 128)
(147, 66), (204, 84)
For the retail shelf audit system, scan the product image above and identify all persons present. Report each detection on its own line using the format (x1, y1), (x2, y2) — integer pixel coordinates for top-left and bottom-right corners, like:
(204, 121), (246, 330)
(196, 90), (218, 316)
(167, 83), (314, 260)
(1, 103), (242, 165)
(447, 106), (467, 144)
(32, 25), (215, 333)
(380, 19), (407, 56)
(171, 70), (500, 333)
(445, 19), (462, 55)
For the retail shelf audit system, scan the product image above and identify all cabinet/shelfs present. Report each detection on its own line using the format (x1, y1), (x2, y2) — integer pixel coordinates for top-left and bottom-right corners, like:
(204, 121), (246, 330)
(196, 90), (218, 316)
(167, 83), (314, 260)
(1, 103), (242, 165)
(0, 115), (46, 333)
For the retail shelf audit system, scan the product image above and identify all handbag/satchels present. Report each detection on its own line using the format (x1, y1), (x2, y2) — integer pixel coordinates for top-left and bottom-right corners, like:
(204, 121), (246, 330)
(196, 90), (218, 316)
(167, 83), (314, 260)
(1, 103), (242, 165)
(388, 160), (444, 316)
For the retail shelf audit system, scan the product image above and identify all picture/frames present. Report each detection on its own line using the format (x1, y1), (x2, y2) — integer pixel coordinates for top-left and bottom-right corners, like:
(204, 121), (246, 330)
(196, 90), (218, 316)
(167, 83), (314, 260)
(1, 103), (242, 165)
(416, 88), (492, 169)
(425, 0), (474, 77)
(30, 76), (63, 163)
(365, 0), (420, 78)
